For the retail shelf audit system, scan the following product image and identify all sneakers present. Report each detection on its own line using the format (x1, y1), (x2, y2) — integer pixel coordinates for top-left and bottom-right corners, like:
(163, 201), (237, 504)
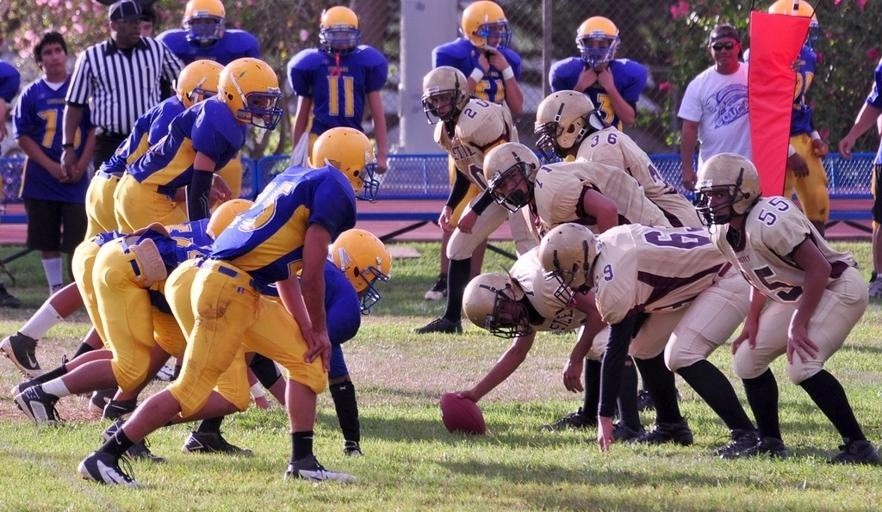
(547, 410), (597, 430)
(10, 380), (65, 427)
(415, 317), (463, 334)
(868, 274), (881, 298)
(78, 451), (138, 487)
(0, 287), (21, 308)
(609, 421), (644, 443)
(101, 404), (138, 422)
(637, 390), (680, 411)
(424, 271), (448, 302)
(828, 437), (879, 465)
(181, 430), (252, 457)
(101, 419), (166, 465)
(285, 458), (356, 483)
(714, 430), (759, 457)
(636, 419), (694, 449)
(0, 332), (42, 377)
(738, 437), (787, 459)
(90, 391), (112, 414)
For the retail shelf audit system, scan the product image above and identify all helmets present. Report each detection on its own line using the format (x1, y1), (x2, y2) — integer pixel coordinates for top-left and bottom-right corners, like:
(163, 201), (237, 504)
(175, 59), (224, 107)
(207, 198), (255, 240)
(692, 153), (761, 227)
(329, 228), (391, 312)
(460, 1), (512, 51)
(318, 6), (361, 56)
(311, 127), (380, 203)
(534, 90), (605, 163)
(483, 143), (540, 214)
(768, 0), (819, 53)
(575, 17), (621, 70)
(422, 66), (468, 125)
(541, 223), (599, 309)
(219, 57), (283, 130)
(183, 0), (228, 41)
(463, 273), (533, 337)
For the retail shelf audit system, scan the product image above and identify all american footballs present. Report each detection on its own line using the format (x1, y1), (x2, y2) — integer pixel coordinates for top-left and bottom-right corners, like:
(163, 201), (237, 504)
(440, 393), (485, 435)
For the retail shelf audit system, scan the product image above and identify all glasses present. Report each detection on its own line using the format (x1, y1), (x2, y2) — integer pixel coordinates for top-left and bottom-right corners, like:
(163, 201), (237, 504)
(709, 41), (738, 52)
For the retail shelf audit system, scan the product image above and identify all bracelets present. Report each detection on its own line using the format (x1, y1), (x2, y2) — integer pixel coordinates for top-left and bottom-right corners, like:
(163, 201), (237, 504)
(787, 144), (795, 157)
(468, 68), (483, 83)
(502, 66), (514, 80)
(811, 131), (822, 143)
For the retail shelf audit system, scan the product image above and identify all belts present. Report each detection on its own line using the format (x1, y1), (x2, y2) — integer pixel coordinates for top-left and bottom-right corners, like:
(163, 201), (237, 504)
(714, 262), (733, 277)
(116, 235), (140, 276)
(219, 265), (255, 286)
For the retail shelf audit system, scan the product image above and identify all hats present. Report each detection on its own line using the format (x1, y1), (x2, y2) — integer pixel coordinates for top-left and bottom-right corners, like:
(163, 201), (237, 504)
(108, 1), (146, 23)
(709, 24), (745, 62)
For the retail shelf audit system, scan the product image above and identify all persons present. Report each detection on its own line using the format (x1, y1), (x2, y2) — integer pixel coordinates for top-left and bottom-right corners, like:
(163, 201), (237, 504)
(695, 151), (882, 468)
(534, 90), (708, 410)
(838, 57), (882, 298)
(457, 243), (645, 442)
(424, 0), (521, 300)
(414, 66), (540, 335)
(482, 141), (675, 233)
(744, 0), (830, 238)
(0, 1), (391, 486)
(678, 25), (802, 192)
(548, 16), (647, 134)
(539, 221), (761, 460)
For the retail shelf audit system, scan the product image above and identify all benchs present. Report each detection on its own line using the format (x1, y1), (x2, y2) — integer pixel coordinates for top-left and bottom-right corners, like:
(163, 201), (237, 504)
(1, 153), (876, 260)
